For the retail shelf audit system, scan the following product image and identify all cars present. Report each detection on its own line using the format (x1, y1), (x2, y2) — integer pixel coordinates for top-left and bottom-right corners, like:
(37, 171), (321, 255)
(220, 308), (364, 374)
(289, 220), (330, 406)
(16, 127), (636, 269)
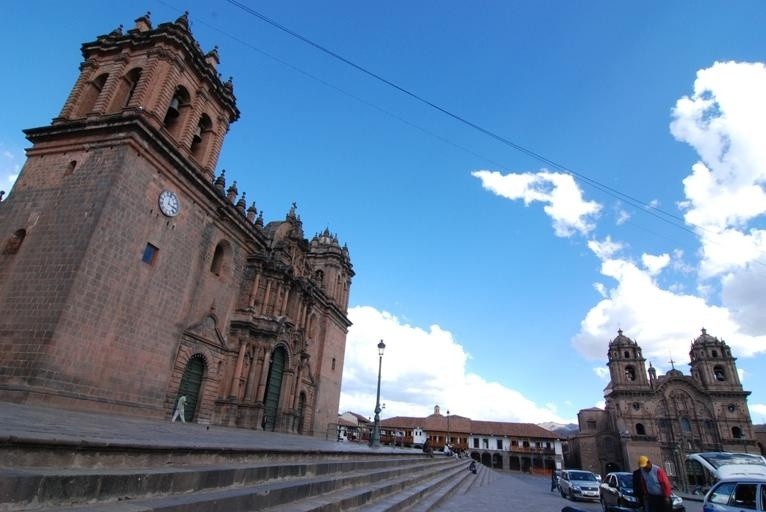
(598, 471), (688, 512)
(552, 469), (602, 502)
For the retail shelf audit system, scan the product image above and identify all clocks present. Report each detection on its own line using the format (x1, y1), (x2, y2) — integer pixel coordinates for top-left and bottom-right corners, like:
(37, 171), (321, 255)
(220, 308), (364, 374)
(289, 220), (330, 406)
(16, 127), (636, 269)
(158, 190), (180, 217)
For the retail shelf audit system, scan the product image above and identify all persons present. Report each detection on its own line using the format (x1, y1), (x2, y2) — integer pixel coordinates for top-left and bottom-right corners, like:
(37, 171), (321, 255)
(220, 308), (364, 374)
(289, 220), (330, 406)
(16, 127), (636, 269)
(469, 461), (478, 474)
(261, 412), (268, 431)
(550, 467), (561, 494)
(422, 438), (434, 458)
(170, 393), (190, 424)
(632, 468), (649, 511)
(444, 444), (470, 462)
(638, 455), (674, 511)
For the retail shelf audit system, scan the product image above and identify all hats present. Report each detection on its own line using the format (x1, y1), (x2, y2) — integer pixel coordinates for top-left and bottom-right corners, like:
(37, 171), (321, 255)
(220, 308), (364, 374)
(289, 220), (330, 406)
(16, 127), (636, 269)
(639, 456), (648, 468)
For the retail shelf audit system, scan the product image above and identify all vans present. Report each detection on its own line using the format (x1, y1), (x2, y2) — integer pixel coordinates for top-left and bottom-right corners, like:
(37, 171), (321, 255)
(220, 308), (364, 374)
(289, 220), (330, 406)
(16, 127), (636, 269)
(685, 452), (766, 512)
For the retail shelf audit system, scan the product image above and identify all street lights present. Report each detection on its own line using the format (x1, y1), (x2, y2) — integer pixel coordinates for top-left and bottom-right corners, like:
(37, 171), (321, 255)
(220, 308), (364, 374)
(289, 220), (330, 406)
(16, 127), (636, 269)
(369, 338), (386, 446)
(445, 407), (450, 446)
